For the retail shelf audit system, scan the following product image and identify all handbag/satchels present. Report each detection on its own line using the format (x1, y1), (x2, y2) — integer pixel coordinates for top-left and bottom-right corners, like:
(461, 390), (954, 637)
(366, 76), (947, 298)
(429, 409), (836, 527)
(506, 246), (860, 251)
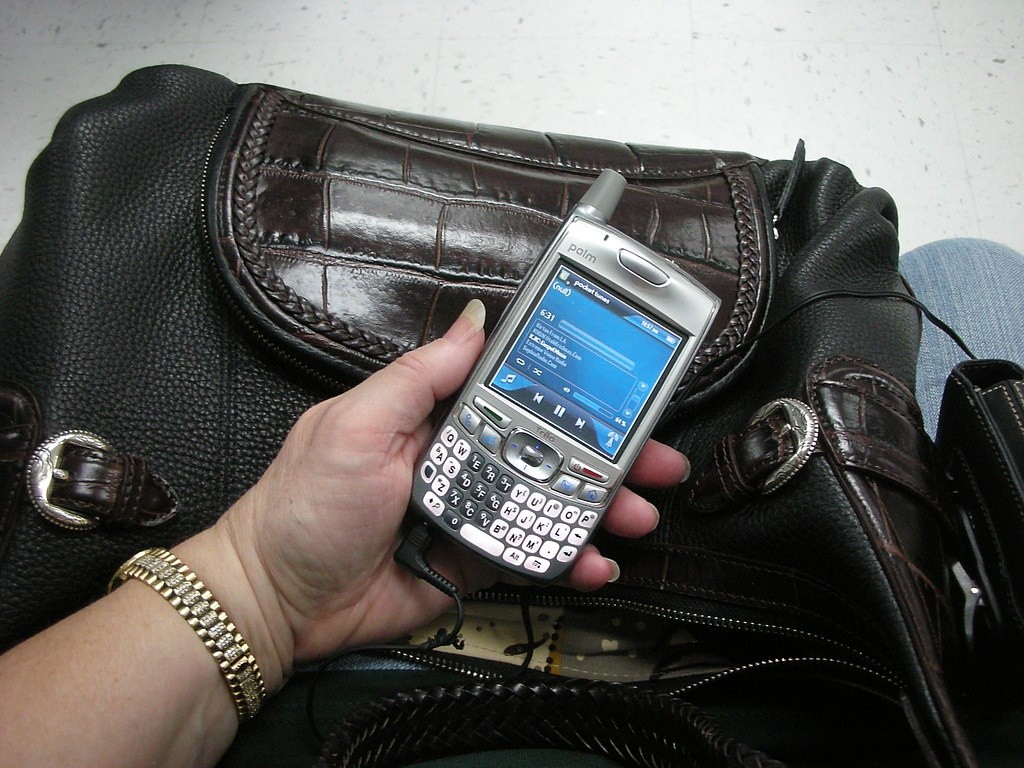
(0, 67), (1024, 768)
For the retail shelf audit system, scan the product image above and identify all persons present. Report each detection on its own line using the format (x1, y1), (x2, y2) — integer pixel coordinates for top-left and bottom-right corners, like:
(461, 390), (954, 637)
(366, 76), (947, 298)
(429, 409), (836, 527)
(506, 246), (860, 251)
(0, 299), (689, 768)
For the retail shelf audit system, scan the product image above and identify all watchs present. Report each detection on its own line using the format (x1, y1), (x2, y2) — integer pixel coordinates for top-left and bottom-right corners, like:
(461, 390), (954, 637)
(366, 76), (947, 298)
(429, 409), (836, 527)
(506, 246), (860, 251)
(109, 546), (266, 727)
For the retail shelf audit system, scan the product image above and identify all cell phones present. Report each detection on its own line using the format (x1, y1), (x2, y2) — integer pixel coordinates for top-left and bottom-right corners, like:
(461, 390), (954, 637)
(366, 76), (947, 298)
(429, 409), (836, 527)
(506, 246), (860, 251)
(411, 169), (722, 584)
